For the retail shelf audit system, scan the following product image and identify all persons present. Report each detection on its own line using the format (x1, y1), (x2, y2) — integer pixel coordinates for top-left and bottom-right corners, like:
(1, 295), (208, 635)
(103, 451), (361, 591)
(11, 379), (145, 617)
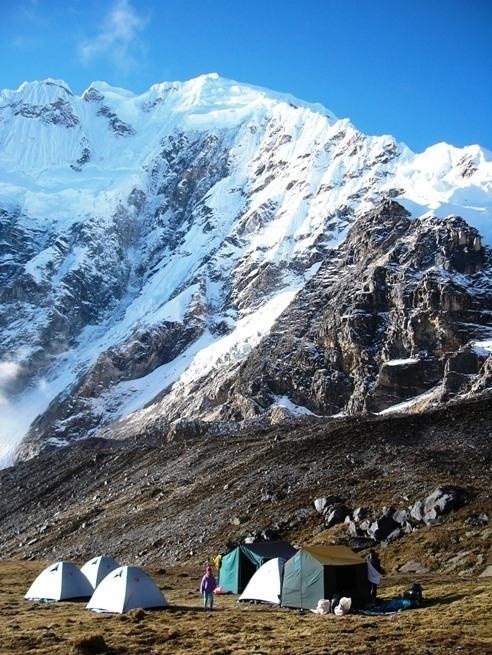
(406, 583), (423, 598)
(366, 549), (380, 597)
(200, 568), (216, 611)
(214, 552), (224, 584)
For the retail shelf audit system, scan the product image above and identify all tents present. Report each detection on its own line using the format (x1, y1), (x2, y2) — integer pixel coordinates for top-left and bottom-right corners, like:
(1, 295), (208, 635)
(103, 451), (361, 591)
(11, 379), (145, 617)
(218, 541), (298, 594)
(281, 544), (370, 611)
(80, 556), (119, 591)
(236, 557), (287, 606)
(86, 566), (169, 614)
(24, 561), (95, 601)
